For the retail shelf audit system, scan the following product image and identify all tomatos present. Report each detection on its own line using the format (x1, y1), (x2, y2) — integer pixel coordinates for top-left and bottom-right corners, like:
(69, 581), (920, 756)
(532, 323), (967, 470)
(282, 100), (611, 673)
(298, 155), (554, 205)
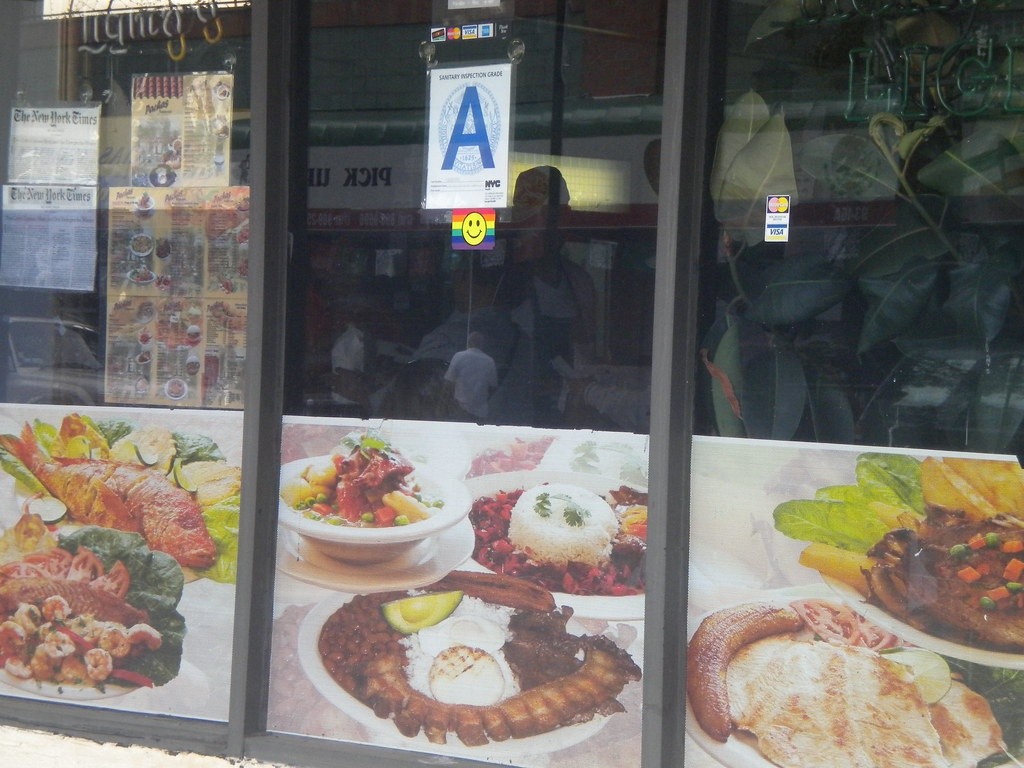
(788, 598), (901, 652)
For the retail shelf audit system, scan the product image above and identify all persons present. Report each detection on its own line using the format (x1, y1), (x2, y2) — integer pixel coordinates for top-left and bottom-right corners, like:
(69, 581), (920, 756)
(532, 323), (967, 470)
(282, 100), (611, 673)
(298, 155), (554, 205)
(435, 333), (497, 423)
(330, 166), (600, 427)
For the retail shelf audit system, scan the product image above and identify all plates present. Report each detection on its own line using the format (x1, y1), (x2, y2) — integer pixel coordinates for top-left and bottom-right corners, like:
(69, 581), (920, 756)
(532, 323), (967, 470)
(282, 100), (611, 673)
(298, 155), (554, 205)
(130, 234), (153, 257)
(133, 196), (154, 211)
(816, 509), (1024, 670)
(686, 599), (953, 768)
(275, 516), (473, 591)
(300, 586), (616, 753)
(148, 161), (177, 188)
(0, 666), (140, 698)
(165, 378), (188, 400)
(135, 352), (153, 365)
(461, 470), (646, 619)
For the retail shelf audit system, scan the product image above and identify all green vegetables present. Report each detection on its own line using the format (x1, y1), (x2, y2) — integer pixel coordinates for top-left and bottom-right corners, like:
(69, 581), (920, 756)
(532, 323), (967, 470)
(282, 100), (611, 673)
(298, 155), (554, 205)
(0, 415), (242, 688)
(535, 492), (592, 527)
(771, 453), (927, 558)
(335, 428), (396, 466)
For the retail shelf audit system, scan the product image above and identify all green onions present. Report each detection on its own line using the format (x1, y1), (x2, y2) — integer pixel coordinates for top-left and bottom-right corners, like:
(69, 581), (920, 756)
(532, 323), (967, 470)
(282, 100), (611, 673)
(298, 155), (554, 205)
(294, 493), (444, 526)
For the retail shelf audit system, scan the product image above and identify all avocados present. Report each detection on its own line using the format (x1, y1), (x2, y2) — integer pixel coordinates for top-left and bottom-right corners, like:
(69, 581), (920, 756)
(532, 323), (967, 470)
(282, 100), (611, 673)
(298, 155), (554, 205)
(377, 589), (464, 633)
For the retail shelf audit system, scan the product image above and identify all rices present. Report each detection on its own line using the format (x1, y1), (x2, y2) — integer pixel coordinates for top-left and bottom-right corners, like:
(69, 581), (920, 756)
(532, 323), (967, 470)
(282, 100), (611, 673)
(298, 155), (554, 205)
(394, 588), (518, 706)
(508, 484), (620, 569)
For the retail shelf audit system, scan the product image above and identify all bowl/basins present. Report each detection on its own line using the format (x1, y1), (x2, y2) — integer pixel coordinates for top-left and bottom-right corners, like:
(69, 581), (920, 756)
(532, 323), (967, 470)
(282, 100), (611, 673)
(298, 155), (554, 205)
(127, 269), (158, 285)
(278, 455), (471, 565)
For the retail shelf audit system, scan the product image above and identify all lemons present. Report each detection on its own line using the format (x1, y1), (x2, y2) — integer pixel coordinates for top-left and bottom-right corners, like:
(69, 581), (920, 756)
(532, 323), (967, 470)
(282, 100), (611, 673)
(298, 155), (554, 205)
(879, 647), (952, 702)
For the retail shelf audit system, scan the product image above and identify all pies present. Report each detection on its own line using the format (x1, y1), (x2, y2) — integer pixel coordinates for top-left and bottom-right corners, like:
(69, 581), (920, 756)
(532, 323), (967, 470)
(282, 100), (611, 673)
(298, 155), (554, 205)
(725, 633), (1008, 767)
